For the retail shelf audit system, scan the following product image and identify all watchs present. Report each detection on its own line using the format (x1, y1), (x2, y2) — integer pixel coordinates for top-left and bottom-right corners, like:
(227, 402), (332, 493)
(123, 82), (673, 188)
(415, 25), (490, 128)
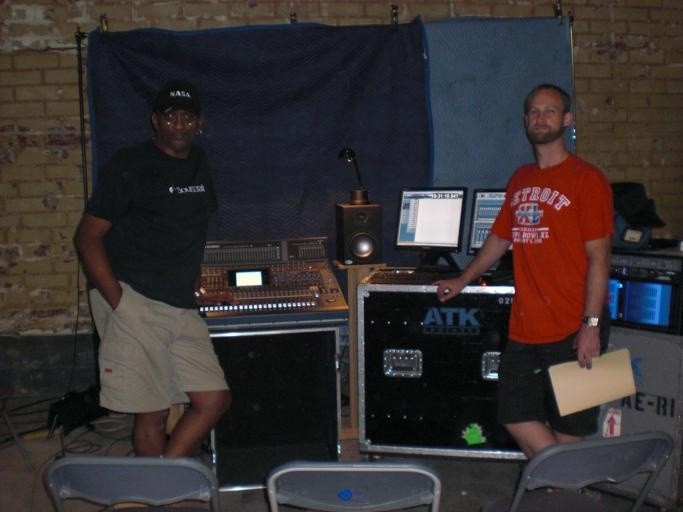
(581, 316), (600, 327)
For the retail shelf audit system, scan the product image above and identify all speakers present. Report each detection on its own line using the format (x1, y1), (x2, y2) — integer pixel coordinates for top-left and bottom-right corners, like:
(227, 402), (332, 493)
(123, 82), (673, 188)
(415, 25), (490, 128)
(334, 199), (383, 265)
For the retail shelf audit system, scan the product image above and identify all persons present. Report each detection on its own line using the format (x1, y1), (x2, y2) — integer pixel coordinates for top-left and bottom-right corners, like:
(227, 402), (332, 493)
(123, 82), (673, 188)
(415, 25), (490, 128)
(430, 84), (614, 491)
(74, 77), (233, 460)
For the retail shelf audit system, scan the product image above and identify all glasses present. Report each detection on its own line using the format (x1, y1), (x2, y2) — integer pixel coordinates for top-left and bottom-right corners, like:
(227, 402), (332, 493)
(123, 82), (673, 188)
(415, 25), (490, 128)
(162, 109), (197, 123)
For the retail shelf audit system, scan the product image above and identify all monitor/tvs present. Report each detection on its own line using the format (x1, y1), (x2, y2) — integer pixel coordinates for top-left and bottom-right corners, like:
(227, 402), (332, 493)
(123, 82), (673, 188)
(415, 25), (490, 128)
(467, 187), (515, 257)
(603, 276), (621, 322)
(623, 277), (674, 332)
(395, 186), (467, 271)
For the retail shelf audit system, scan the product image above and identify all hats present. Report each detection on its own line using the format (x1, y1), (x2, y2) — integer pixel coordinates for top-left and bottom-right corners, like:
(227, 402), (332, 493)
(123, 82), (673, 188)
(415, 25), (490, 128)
(154, 83), (204, 114)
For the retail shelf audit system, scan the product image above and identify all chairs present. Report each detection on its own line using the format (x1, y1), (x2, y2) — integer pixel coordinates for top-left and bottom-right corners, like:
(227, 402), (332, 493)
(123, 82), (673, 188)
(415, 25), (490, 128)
(42, 453), (220, 511)
(267, 459), (443, 511)
(481, 429), (675, 511)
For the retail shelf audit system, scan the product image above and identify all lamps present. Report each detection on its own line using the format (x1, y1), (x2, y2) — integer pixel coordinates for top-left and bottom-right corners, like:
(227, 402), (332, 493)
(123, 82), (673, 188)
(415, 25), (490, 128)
(335, 146), (369, 206)
